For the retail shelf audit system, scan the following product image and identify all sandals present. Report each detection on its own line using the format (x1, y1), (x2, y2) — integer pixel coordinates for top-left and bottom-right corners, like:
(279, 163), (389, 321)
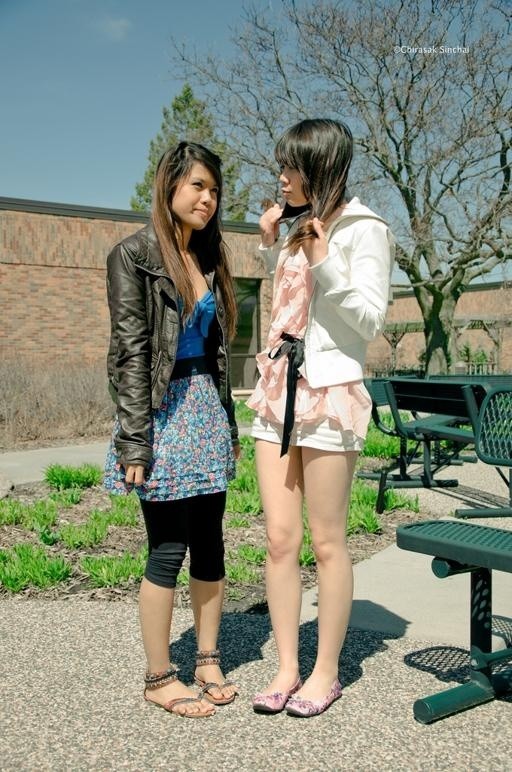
(144, 663), (216, 717)
(193, 648), (235, 705)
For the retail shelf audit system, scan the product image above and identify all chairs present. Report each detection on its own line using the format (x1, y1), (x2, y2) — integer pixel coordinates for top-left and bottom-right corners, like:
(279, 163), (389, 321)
(364, 375), (512, 726)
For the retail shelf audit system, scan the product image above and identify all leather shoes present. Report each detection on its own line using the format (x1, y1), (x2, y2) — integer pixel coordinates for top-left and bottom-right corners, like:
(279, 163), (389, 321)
(253, 673), (302, 712)
(285, 678), (342, 717)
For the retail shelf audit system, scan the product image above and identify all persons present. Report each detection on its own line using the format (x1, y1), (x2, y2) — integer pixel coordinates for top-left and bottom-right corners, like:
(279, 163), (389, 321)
(103, 139), (241, 719)
(251, 117), (402, 720)
